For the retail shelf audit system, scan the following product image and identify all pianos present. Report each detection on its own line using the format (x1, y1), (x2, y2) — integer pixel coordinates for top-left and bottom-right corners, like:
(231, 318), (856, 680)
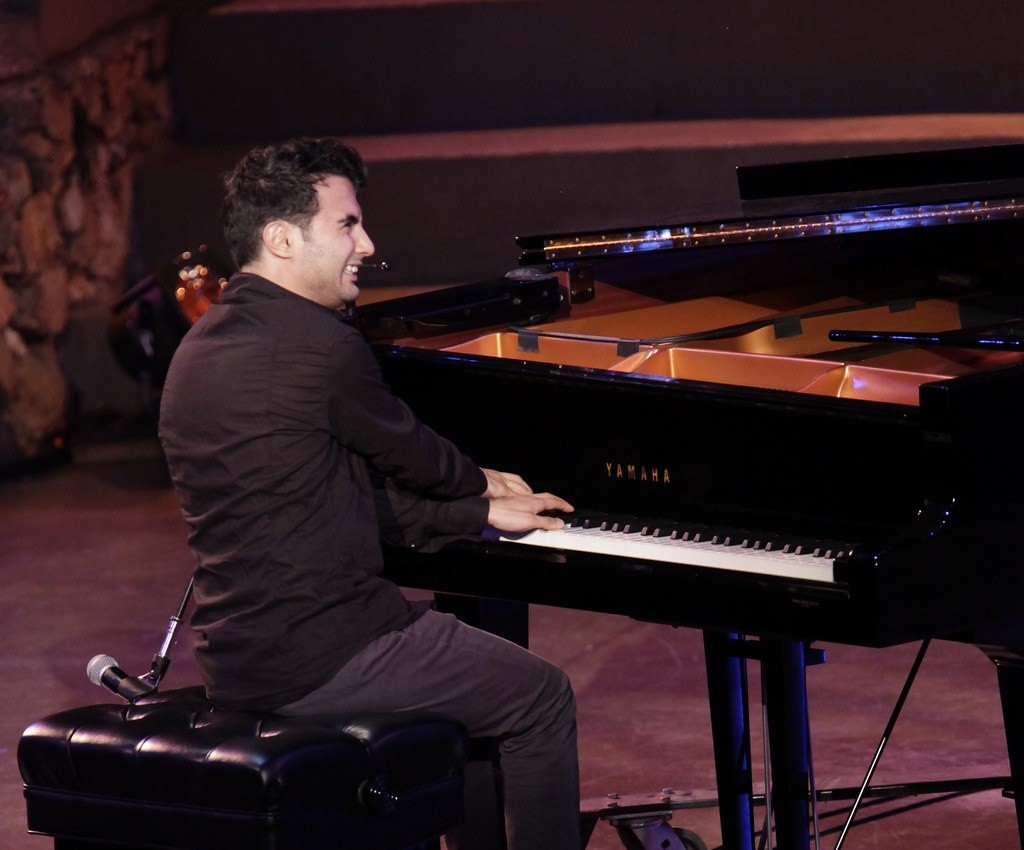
(338, 139), (1024, 850)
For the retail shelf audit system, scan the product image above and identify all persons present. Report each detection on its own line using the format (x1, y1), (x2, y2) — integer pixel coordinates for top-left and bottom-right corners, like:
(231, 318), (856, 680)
(157, 137), (605, 850)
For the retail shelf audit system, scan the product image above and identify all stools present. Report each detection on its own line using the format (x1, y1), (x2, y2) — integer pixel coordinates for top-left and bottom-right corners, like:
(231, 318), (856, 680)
(18, 685), (469, 850)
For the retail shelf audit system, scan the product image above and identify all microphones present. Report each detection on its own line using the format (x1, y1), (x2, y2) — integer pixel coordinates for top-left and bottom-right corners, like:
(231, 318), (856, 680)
(110, 238), (213, 314)
(86, 653), (153, 703)
